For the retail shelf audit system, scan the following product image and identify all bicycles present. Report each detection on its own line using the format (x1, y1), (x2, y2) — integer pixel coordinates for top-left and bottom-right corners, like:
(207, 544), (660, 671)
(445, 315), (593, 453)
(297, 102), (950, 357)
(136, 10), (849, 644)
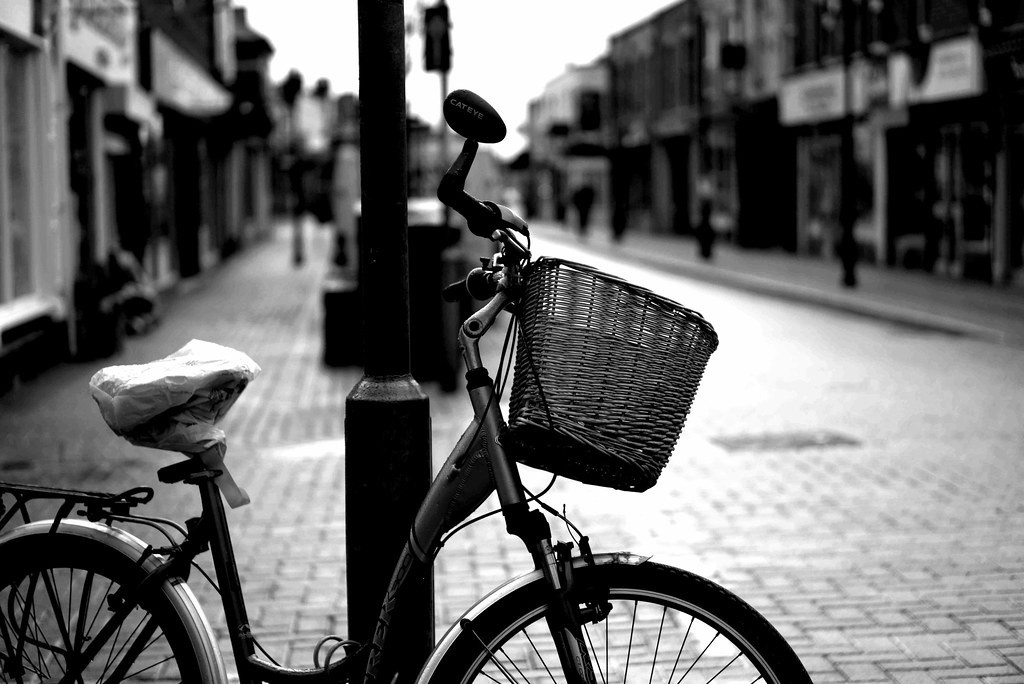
(0, 89), (812, 684)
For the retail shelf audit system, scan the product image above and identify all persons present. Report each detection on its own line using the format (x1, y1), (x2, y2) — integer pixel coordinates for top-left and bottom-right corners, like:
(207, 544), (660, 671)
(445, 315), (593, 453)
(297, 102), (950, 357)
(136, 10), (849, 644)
(572, 182), (595, 232)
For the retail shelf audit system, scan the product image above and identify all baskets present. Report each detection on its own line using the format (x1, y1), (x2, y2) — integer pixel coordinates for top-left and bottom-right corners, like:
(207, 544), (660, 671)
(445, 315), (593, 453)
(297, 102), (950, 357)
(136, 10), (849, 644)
(507, 256), (719, 493)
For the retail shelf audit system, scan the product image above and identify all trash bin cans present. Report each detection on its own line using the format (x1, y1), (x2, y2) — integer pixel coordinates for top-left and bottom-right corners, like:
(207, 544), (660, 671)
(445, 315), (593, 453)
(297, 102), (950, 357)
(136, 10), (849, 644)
(352, 223), (465, 385)
